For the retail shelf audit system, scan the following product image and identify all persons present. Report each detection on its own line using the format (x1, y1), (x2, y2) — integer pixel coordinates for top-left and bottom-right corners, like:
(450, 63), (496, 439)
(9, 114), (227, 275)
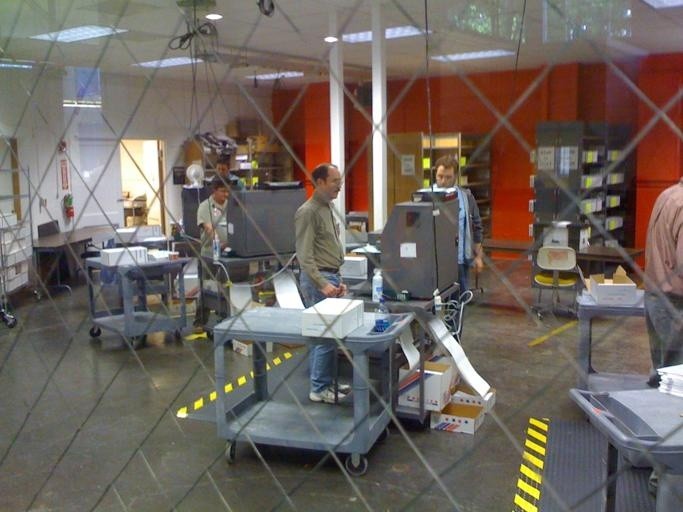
(293, 162), (351, 404)
(432, 156), (484, 342)
(192, 178), (232, 334)
(210, 157), (245, 191)
(643, 177), (683, 388)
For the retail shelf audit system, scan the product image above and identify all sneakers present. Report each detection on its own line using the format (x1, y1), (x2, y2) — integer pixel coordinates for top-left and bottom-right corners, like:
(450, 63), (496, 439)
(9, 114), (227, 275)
(309, 380), (352, 405)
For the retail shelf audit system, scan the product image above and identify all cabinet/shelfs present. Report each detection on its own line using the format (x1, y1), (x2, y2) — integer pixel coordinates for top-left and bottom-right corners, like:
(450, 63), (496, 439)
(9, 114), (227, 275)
(185, 140), (294, 189)
(529, 122), (632, 290)
(368, 132), (492, 256)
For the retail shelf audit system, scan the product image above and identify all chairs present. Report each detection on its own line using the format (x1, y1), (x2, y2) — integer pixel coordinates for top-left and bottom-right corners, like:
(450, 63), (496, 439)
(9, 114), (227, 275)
(530, 247), (580, 320)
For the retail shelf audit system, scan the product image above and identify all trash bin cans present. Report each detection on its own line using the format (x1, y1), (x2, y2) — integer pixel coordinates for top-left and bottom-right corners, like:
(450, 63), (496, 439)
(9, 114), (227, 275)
(346, 215), (368, 233)
(124, 207), (142, 227)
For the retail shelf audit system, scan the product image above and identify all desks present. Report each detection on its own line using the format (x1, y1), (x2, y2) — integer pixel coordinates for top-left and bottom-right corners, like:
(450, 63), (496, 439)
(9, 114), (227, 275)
(33, 224), (117, 296)
(577, 247), (644, 276)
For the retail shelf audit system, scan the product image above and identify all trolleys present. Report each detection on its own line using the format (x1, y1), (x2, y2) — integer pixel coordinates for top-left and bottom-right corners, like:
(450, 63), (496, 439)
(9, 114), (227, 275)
(83, 255), (192, 349)
(341, 280), (460, 431)
(198, 249), (295, 339)
(567, 386), (683, 511)
(0, 225), (24, 329)
(211, 306), (413, 475)
(573, 288), (649, 423)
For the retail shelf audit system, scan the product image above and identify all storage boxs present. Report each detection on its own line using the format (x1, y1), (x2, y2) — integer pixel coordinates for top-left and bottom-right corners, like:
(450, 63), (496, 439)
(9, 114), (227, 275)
(100, 247), (148, 266)
(584, 266), (636, 304)
(227, 115), (264, 139)
(396, 305), (496, 435)
(233, 302), (272, 356)
(301, 298), (364, 339)
(116, 225), (161, 243)
(339, 254), (367, 276)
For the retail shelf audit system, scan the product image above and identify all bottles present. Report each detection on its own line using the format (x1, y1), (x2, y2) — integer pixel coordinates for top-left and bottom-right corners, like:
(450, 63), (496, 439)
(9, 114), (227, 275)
(373, 297), (391, 332)
(371, 270), (383, 302)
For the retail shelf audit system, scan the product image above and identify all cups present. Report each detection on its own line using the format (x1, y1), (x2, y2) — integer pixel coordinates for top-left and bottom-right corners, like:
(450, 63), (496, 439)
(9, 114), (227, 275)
(168, 251), (179, 262)
(212, 240), (220, 261)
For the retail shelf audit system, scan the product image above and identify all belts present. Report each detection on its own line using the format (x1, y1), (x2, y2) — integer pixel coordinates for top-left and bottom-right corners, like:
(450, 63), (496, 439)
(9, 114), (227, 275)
(650, 291), (683, 301)
